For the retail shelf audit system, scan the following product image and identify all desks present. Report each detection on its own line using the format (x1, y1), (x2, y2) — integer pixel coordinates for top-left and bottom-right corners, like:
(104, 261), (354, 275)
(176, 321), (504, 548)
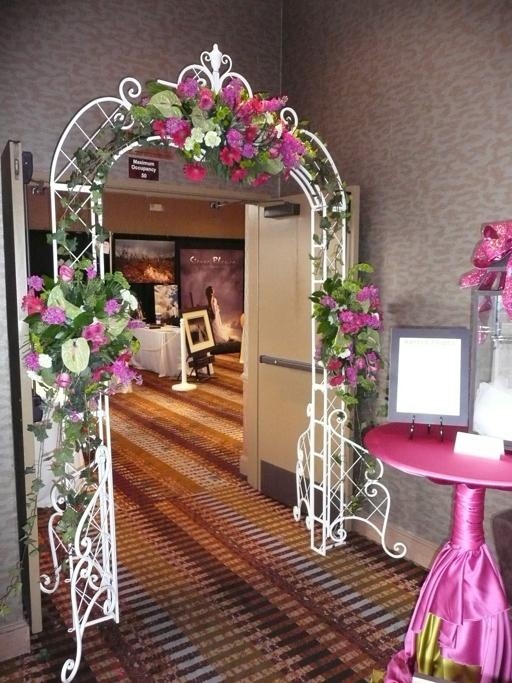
(363, 421), (511, 683)
(127, 323), (181, 375)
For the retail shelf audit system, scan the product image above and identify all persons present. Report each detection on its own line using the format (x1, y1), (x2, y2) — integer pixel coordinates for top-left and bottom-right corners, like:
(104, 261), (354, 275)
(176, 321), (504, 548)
(204, 285), (234, 343)
(197, 321), (206, 343)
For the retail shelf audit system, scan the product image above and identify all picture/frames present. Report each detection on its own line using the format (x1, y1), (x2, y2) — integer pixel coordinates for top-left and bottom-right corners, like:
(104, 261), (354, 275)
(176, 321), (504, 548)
(180, 305), (216, 355)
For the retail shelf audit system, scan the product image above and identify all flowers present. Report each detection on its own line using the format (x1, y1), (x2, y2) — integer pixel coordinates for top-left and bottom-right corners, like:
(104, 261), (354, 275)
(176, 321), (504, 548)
(309, 259), (393, 516)
(1, 261), (146, 617)
(42, 71), (353, 272)
